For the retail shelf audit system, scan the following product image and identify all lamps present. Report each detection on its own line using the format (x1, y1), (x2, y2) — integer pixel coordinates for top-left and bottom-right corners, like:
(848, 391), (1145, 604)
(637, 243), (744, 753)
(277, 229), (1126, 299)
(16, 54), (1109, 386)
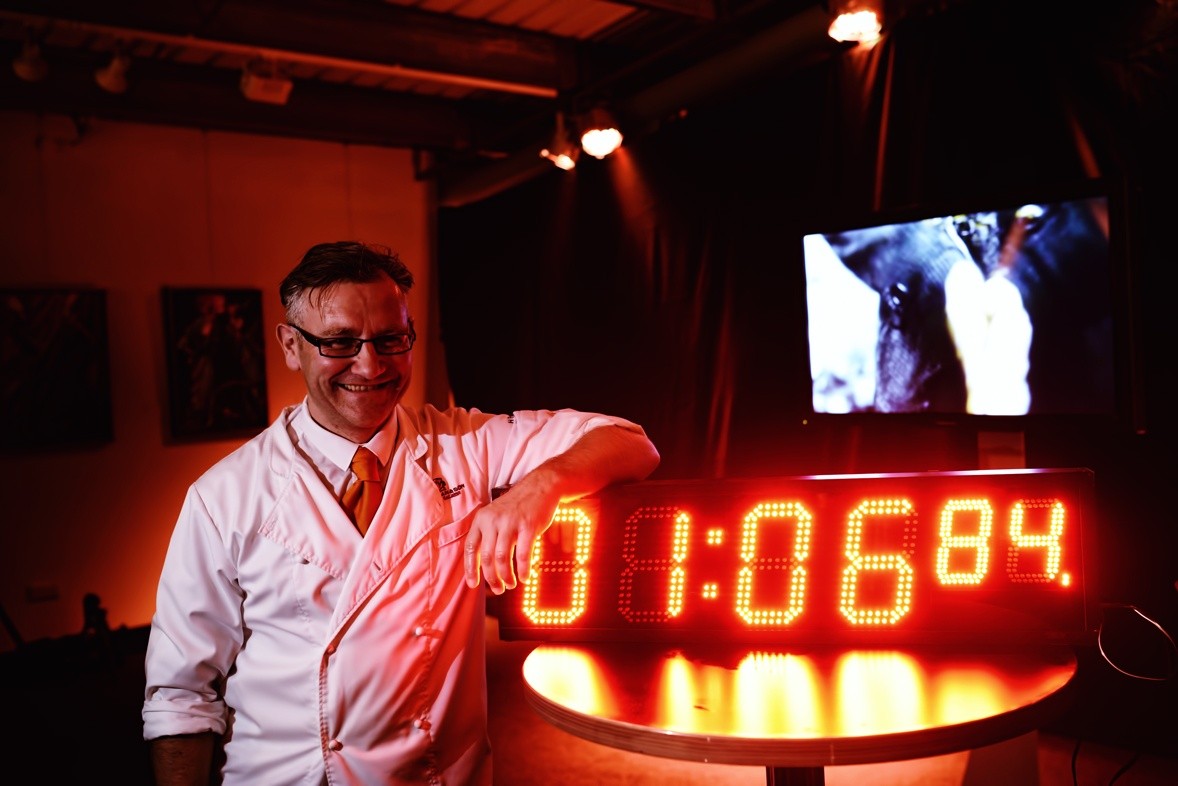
(89, 51), (131, 97)
(825, 0), (885, 51)
(529, 96), (623, 172)
(15, 36), (52, 81)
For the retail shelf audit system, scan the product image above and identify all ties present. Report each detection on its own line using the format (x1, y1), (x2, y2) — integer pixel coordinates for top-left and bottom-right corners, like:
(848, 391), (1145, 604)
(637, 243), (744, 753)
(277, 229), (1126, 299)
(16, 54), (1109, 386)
(340, 448), (385, 538)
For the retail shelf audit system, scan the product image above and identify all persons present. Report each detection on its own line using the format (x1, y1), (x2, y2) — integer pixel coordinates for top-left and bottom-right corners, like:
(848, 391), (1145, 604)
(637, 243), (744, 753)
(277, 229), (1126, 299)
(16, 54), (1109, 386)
(142, 240), (660, 786)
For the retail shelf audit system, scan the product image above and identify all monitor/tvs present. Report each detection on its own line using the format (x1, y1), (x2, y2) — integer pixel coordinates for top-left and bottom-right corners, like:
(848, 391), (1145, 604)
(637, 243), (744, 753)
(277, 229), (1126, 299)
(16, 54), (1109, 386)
(792, 172), (1149, 439)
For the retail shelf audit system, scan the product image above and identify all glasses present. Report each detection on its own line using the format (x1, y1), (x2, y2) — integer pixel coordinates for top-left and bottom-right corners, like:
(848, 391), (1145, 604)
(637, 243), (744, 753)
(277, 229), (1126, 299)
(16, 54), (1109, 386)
(288, 320), (416, 357)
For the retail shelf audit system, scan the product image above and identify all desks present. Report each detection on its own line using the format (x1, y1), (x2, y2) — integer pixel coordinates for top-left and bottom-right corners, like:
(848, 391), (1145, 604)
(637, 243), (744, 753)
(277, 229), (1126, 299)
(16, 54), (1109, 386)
(519, 644), (1077, 785)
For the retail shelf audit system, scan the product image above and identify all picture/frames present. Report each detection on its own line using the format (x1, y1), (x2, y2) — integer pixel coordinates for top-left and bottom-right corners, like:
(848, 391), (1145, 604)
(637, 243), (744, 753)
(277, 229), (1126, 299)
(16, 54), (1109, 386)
(2, 287), (115, 449)
(164, 288), (270, 439)
